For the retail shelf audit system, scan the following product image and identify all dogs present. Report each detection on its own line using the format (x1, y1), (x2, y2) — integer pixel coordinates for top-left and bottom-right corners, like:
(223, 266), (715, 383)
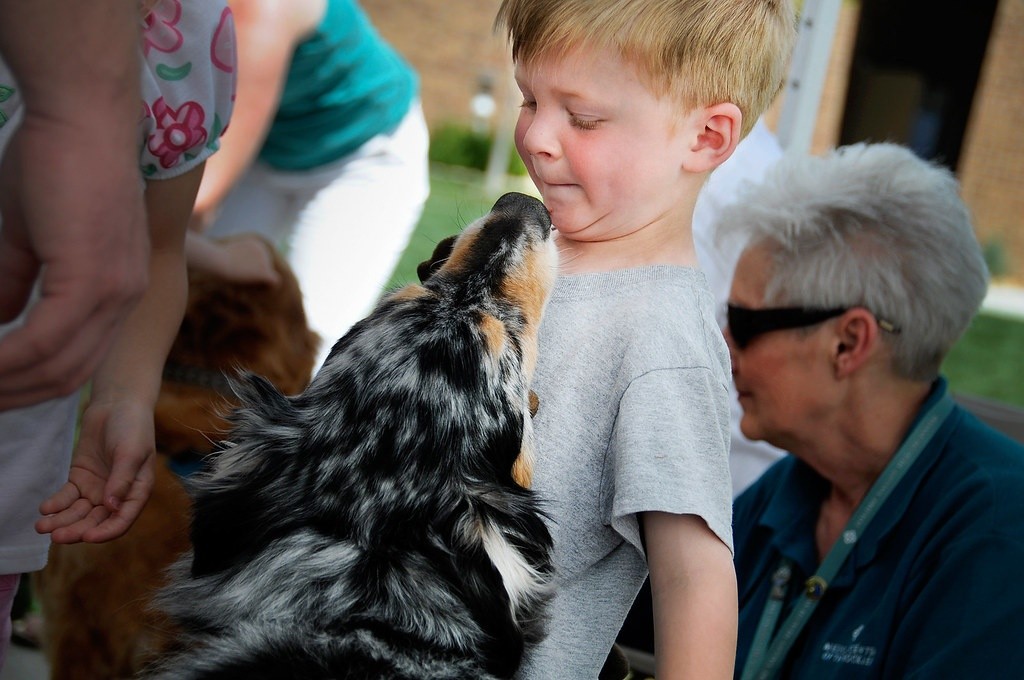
(40, 190), (563, 680)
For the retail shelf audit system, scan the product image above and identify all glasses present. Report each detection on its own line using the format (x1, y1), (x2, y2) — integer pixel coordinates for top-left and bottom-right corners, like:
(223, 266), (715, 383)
(727, 303), (902, 353)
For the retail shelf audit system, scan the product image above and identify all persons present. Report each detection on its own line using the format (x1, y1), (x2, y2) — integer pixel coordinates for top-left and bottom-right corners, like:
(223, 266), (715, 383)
(189, 0), (432, 383)
(600, 138), (1024, 680)
(482, 0), (799, 680)
(0, 0), (236, 679)
